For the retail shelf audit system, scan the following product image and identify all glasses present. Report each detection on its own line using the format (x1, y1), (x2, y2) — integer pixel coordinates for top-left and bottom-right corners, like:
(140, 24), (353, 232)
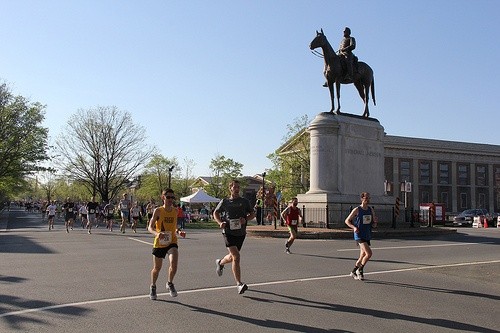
(166, 196), (175, 199)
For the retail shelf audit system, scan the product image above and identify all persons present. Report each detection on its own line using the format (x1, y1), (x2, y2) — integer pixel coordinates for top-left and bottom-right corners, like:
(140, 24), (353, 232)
(344, 191), (378, 281)
(255, 197), (262, 225)
(14, 200), (24, 207)
(281, 198), (304, 255)
(61, 196), (115, 234)
(176, 203), (211, 229)
(117, 193), (158, 233)
(24, 200), (61, 231)
(213, 180), (256, 293)
(322, 27), (359, 87)
(148, 188), (187, 300)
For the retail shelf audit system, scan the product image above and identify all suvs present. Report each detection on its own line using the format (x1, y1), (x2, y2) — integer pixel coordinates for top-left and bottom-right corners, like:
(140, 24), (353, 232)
(453, 209), (493, 227)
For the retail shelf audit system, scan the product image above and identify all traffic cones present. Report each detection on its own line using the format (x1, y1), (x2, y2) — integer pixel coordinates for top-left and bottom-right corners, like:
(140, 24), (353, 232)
(483, 218), (488, 228)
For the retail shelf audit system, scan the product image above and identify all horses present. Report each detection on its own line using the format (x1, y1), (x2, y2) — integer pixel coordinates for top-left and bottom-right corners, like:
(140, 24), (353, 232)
(309, 29), (376, 117)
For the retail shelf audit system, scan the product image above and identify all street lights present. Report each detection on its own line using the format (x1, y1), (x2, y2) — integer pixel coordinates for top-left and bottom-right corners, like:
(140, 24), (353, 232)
(261, 172), (267, 226)
(168, 165), (175, 188)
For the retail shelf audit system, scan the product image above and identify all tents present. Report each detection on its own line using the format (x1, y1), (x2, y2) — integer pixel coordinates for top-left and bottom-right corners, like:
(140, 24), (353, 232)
(179, 189), (221, 215)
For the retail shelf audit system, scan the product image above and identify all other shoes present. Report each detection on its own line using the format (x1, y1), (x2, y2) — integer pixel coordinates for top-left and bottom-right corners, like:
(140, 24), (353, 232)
(215, 259), (224, 277)
(150, 285), (157, 300)
(356, 268), (364, 281)
(237, 282), (248, 294)
(166, 281), (177, 297)
(350, 271), (359, 280)
(285, 250), (292, 255)
(285, 241), (288, 249)
